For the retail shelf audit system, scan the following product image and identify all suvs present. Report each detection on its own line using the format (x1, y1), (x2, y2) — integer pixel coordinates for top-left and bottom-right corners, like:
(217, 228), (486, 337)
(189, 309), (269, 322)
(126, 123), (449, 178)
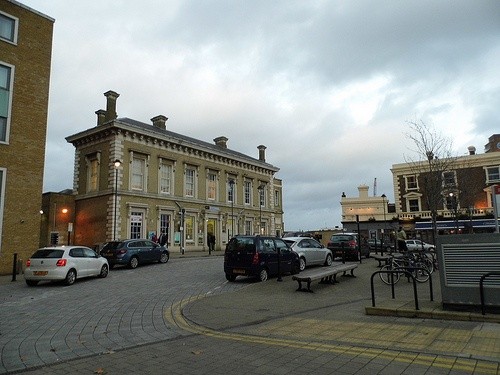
(223, 234), (300, 284)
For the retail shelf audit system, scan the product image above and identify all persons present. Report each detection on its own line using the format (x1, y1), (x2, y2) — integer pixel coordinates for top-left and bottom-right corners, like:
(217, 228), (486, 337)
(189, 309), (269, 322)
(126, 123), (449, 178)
(397, 225), (409, 254)
(158, 231), (167, 247)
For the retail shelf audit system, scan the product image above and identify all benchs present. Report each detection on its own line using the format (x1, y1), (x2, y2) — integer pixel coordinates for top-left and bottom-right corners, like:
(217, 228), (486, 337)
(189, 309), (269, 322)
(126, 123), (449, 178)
(292, 264), (358, 293)
(375, 256), (403, 267)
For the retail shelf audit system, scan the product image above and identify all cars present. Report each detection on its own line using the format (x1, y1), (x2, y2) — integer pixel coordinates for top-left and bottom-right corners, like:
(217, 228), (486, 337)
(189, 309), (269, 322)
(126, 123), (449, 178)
(327, 232), (371, 262)
(98, 238), (170, 271)
(23, 245), (109, 288)
(280, 236), (333, 273)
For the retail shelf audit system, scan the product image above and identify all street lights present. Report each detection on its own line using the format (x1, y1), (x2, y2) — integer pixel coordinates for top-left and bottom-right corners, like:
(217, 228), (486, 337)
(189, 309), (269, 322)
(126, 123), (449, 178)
(175, 201), (183, 256)
(380, 193), (388, 229)
(113, 159), (121, 239)
(256, 183), (267, 235)
(356, 214), (364, 264)
(62, 208), (71, 246)
(228, 180), (236, 237)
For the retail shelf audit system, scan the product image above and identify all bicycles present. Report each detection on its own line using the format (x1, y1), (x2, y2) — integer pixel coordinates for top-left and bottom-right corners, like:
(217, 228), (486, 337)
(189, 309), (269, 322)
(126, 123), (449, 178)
(375, 242), (438, 288)
